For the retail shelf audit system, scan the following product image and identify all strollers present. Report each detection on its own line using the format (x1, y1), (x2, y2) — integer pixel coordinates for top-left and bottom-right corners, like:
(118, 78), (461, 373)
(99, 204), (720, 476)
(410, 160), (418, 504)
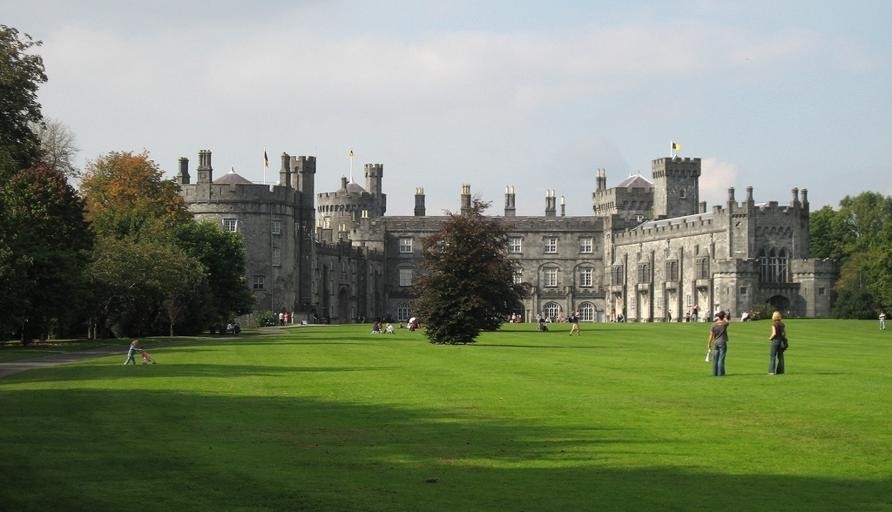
(137, 346), (156, 367)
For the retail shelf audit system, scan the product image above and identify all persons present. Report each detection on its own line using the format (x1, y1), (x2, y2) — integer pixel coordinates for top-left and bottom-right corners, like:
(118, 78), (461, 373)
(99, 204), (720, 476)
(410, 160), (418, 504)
(506, 310), (582, 336)
(768, 310), (785, 375)
(707, 311), (730, 376)
(223, 310), (306, 337)
(611, 305), (617, 322)
(878, 311), (886, 331)
(357, 312), (422, 336)
(667, 304), (752, 324)
(606, 304), (611, 322)
(122, 339), (145, 368)
(616, 312), (624, 323)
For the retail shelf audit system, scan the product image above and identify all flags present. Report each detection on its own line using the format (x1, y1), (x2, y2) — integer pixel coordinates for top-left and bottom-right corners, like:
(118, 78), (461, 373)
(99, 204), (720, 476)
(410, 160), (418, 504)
(264, 151), (269, 168)
(672, 142), (680, 150)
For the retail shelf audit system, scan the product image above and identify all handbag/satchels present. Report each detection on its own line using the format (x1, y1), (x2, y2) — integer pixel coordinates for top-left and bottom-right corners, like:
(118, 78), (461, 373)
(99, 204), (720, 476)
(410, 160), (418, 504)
(779, 338), (788, 350)
